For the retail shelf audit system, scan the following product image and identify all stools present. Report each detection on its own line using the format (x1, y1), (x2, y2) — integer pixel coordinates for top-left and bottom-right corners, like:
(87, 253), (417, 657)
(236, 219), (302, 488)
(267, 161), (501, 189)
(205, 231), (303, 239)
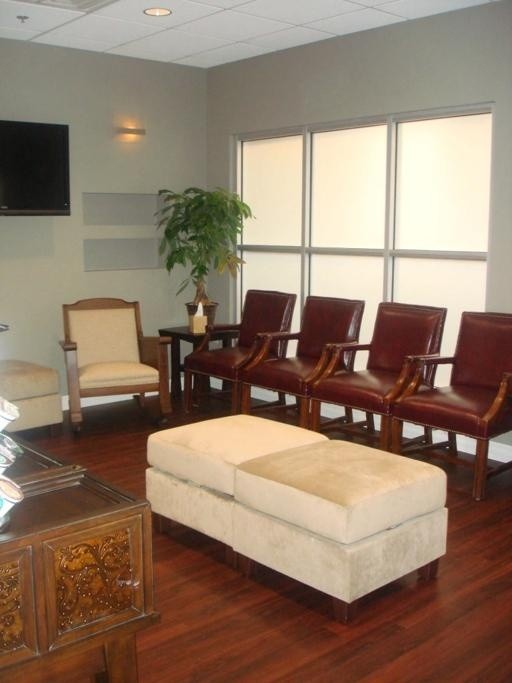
(0, 357), (64, 438)
(231, 440), (450, 626)
(146, 411), (330, 571)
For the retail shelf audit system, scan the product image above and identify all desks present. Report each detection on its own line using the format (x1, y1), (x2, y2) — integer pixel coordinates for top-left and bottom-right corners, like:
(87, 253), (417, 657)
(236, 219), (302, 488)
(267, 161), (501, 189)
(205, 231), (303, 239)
(0, 437), (163, 683)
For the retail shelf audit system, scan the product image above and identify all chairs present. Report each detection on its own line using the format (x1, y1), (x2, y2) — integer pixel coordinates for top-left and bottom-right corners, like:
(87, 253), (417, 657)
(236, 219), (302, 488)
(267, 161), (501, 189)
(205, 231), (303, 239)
(391, 311), (512, 500)
(306, 302), (446, 453)
(58, 296), (175, 440)
(238, 297), (365, 430)
(184, 290), (297, 421)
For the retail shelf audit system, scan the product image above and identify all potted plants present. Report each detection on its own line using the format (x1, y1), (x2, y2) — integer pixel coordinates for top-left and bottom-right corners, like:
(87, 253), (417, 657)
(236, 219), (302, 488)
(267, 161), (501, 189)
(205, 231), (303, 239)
(153, 185), (256, 327)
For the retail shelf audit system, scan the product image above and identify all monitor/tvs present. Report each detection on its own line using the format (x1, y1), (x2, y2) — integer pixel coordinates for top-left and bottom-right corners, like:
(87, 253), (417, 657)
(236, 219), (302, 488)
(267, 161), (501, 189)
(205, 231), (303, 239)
(0, 119), (72, 216)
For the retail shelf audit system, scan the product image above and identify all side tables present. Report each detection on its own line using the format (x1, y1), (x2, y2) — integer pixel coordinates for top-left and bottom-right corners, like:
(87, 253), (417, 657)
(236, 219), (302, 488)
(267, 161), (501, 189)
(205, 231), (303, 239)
(158, 324), (240, 407)
